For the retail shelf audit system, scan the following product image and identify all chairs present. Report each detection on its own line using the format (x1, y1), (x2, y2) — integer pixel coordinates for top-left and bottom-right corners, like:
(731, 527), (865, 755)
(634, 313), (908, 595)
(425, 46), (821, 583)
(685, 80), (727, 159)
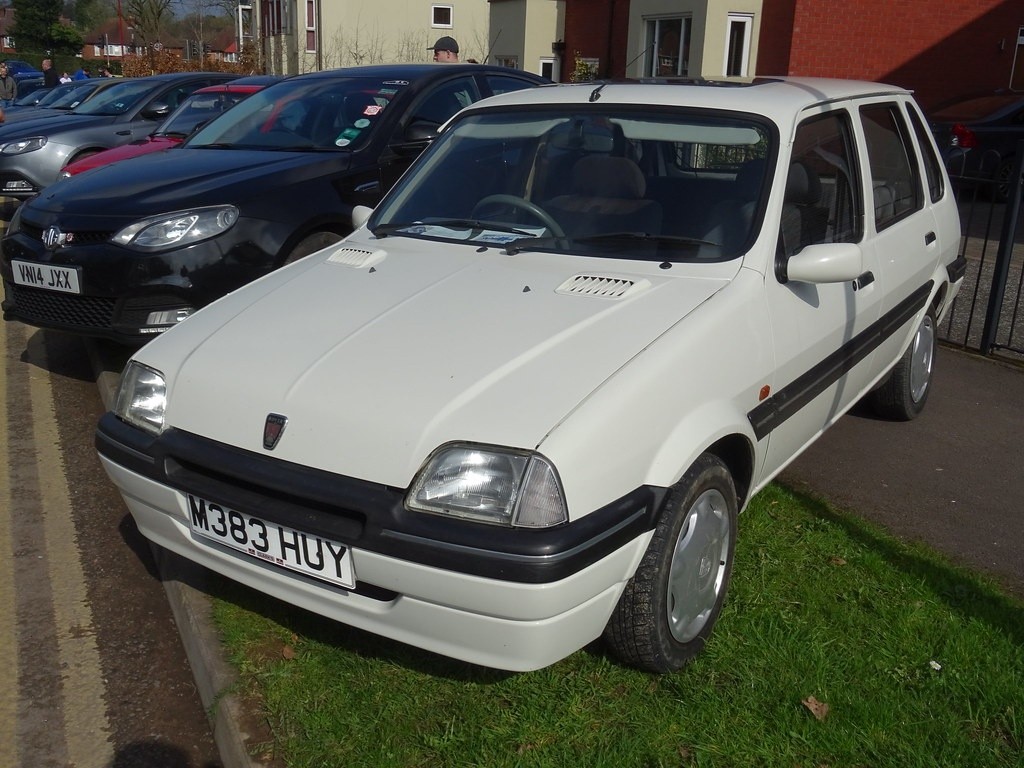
(303, 97), (339, 143)
(722, 155), (829, 257)
(540, 154), (665, 254)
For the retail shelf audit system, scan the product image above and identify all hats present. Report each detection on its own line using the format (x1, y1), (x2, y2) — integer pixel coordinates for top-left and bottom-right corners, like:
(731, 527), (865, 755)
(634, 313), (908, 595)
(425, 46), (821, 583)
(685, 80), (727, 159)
(427, 36), (459, 53)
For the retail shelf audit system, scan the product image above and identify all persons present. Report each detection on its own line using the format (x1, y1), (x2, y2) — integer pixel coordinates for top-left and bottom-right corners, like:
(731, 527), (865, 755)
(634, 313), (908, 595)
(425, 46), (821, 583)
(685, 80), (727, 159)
(944, 122), (993, 203)
(433, 36), (461, 65)
(0, 62), (18, 123)
(42, 58), (116, 89)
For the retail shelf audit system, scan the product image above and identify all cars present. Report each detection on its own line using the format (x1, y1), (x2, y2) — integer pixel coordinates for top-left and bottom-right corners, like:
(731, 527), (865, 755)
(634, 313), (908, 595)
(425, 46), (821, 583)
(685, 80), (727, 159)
(924, 94), (1024, 203)
(95, 41), (968, 675)
(1, 57), (284, 200)
(0, 27), (640, 342)
